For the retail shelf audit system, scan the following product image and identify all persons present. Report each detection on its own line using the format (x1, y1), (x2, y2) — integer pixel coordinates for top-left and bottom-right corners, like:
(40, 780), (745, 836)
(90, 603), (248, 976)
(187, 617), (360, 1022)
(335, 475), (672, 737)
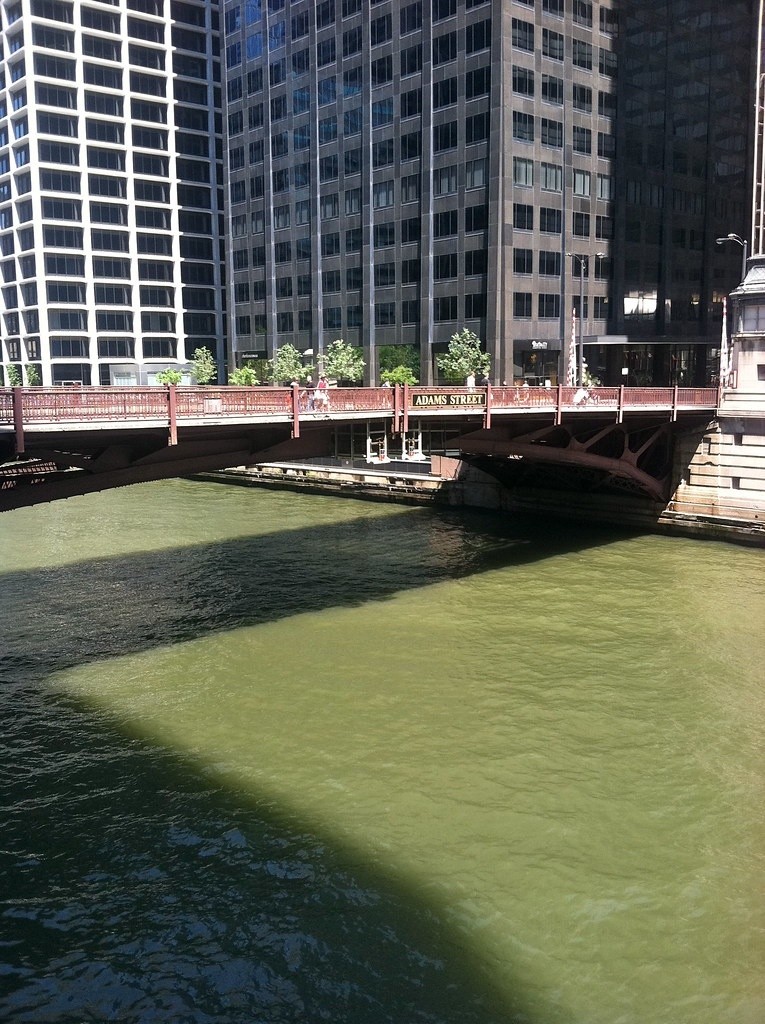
(594, 377), (604, 387)
(481, 373), (491, 386)
(381, 378), (392, 410)
(522, 380), (530, 404)
(466, 371), (476, 387)
(290, 373), (331, 417)
(501, 380), (508, 389)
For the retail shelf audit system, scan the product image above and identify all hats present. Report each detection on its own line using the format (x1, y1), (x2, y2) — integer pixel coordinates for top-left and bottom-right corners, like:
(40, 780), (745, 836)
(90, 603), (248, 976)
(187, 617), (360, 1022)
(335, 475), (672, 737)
(319, 373), (326, 379)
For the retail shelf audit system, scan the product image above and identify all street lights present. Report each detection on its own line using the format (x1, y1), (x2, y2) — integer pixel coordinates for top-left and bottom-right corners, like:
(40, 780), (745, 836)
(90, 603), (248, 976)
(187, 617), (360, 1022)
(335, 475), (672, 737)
(717, 233), (747, 283)
(566, 251), (604, 387)
